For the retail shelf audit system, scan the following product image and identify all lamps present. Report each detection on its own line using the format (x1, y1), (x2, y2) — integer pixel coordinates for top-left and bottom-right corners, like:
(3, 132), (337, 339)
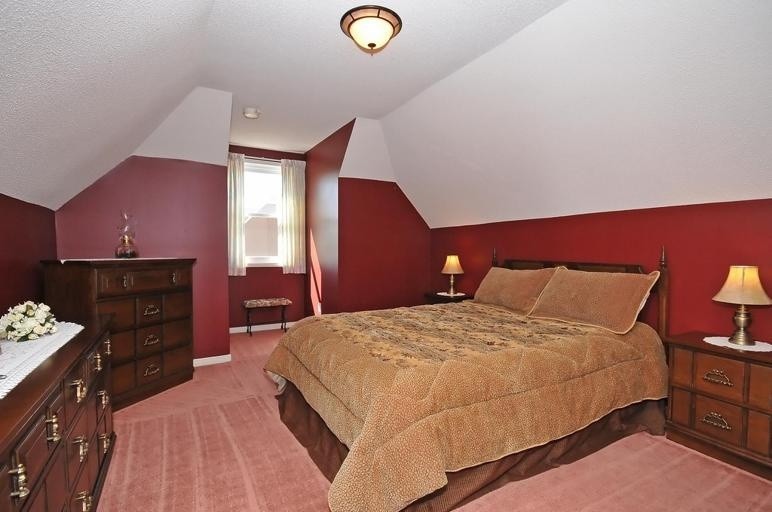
(711, 265), (772, 345)
(339, 4), (402, 51)
(440, 255), (465, 294)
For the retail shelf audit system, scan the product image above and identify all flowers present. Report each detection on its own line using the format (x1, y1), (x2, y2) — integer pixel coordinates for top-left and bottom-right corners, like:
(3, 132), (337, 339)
(0, 297), (58, 343)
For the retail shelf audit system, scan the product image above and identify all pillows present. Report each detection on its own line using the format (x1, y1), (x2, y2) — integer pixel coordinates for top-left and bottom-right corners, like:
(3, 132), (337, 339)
(469, 265), (661, 335)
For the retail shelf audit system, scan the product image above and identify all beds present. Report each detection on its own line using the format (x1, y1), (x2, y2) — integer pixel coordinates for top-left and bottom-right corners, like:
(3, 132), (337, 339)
(261, 246), (671, 512)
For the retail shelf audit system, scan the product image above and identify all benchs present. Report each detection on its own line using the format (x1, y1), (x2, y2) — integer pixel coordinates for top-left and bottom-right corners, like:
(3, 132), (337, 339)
(243, 297), (293, 337)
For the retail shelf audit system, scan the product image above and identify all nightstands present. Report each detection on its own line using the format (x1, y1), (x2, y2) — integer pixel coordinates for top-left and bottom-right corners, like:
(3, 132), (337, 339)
(663, 330), (772, 482)
(424, 292), (474, 304)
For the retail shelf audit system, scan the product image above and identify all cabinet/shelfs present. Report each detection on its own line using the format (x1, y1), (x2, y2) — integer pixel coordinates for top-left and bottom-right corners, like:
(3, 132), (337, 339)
(39, 257), (198, 413)
(0, 312), (116, 512)
